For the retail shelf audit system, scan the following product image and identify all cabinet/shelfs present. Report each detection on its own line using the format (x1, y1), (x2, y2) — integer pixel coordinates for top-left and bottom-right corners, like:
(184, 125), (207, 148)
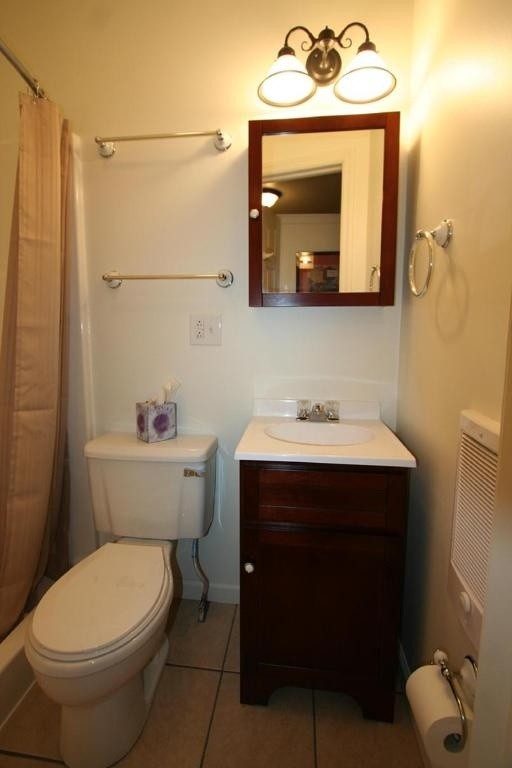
(239, 463), (409, 724)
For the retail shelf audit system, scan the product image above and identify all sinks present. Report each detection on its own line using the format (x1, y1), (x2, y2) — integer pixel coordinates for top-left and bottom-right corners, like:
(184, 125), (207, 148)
(264, 422), (375, 447)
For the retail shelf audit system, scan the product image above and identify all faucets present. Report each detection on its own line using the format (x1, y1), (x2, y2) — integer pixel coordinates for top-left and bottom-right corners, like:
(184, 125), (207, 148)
(308, 403), (326, 422)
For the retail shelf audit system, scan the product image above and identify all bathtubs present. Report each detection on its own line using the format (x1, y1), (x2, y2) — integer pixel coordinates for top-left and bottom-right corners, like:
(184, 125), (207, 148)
(0, 604), (38, 731)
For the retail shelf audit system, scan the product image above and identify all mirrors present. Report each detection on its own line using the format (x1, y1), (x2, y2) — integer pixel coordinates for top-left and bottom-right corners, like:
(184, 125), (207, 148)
(243, 109), (400, 308)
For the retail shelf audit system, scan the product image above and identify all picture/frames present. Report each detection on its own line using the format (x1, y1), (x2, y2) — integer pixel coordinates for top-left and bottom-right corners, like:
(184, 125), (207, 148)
(296, 250), (340, 293)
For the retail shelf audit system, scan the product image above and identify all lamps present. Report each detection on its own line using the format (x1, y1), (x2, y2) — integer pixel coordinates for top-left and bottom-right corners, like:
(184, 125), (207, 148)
(261, 187), (282, 208)
(254, 20), (397, 108)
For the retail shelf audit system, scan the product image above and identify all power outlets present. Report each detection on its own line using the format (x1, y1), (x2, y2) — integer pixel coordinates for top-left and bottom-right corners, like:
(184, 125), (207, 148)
(190, 316), (221, 346)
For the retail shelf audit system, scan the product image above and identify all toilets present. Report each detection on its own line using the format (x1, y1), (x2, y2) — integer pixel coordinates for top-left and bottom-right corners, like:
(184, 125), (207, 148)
(24, 433), (218, 768)
